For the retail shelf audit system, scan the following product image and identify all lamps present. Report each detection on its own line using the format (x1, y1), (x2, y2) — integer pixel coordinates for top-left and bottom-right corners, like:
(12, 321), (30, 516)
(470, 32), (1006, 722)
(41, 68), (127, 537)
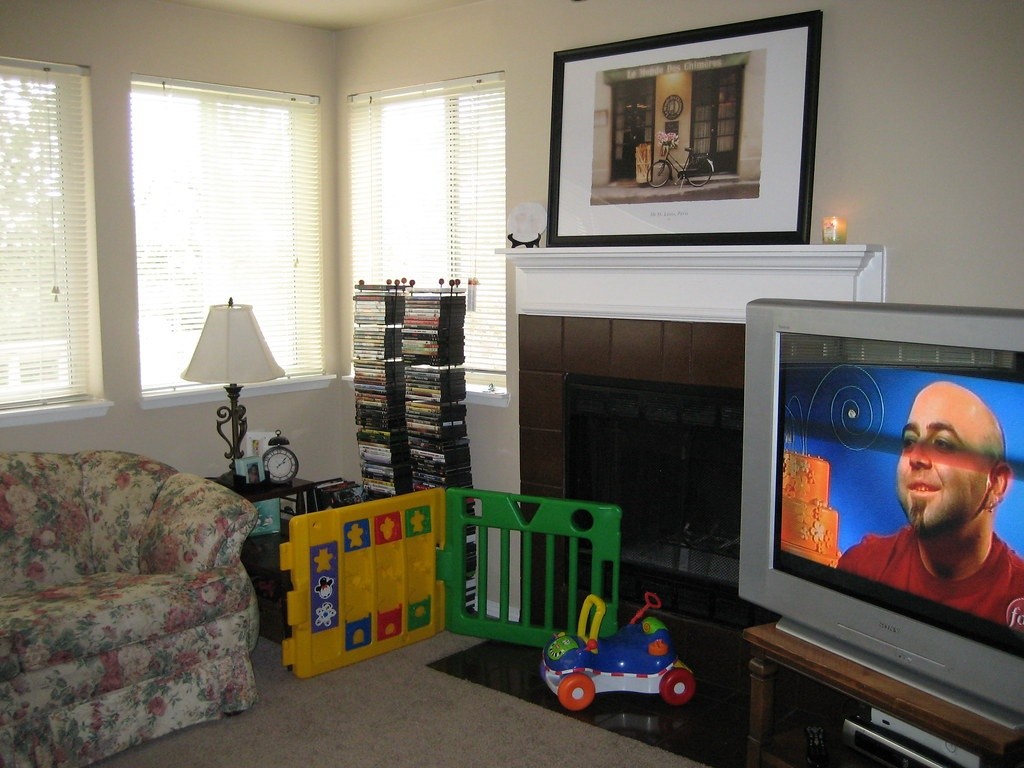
(179, 298), (286, 492)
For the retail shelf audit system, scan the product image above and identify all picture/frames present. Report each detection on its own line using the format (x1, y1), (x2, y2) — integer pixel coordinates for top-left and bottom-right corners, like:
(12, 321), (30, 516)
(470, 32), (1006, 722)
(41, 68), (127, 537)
(235, 456), (265, 484)
(541, 9), (823, 248)
(245, 431), (276, 472)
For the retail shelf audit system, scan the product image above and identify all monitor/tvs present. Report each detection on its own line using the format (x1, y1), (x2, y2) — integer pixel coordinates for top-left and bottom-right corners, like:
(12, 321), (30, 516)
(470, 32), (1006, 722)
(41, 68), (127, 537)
(736, 297), (1024, 732)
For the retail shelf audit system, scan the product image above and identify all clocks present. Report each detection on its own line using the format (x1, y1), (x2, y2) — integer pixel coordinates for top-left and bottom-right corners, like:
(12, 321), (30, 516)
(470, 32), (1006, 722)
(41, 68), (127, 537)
(263, 427), (299, 485)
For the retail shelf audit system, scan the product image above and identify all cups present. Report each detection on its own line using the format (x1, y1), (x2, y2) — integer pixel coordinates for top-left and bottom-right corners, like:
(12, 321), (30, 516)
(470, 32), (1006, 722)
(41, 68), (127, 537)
(822, 216), (847, 244)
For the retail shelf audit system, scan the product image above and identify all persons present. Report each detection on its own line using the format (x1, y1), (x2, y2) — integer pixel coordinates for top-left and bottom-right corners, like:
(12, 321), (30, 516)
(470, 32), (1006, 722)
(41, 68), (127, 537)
(249, 465), (258, 482)
(836, 382), (1024, 632)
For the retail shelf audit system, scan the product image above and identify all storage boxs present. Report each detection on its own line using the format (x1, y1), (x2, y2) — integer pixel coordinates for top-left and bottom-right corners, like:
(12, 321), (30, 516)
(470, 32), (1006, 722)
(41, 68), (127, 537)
(248, 497), (281, 535)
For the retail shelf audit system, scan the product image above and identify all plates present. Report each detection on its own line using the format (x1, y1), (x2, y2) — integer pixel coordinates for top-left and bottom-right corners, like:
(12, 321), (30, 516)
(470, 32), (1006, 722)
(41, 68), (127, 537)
(507, 202), (547, 242)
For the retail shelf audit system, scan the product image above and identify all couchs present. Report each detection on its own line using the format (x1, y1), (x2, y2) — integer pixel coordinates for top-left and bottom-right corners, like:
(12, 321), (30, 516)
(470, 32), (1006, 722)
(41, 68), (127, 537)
(0, 447), (261, 768)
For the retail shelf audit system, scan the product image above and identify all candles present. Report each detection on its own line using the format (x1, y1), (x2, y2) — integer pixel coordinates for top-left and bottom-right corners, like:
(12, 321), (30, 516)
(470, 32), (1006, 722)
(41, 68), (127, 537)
(822, 216), (848, 245)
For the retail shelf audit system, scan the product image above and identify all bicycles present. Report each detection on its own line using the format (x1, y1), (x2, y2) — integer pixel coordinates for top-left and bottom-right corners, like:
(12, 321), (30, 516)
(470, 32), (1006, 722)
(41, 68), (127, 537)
(646, 142), (716, 191)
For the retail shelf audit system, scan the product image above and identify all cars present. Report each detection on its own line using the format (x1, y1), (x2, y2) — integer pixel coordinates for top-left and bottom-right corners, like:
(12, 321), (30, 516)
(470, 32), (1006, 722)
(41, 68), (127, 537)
(540, 595), (698, 714)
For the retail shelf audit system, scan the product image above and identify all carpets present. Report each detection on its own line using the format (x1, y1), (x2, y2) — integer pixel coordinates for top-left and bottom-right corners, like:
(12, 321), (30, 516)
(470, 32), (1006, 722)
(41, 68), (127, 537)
(426, 637), (751, 768)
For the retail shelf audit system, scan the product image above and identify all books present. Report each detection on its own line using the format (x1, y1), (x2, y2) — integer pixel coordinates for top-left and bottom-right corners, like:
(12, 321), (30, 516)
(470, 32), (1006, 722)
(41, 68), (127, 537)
(351, 285), (473, 496)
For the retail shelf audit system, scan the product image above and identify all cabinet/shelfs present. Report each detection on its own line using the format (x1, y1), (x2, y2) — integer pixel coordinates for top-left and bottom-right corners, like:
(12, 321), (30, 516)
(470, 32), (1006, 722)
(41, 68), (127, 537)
(734, 622), (1024, 768)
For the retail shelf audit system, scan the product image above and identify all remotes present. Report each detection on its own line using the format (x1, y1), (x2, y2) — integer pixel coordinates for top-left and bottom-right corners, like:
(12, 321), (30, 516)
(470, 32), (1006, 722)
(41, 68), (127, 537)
(804, 722), (829, 768)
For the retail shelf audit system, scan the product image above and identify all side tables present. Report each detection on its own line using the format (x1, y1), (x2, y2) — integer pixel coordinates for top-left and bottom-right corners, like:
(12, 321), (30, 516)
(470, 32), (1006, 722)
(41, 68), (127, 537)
(209, 476), (317, 645)
